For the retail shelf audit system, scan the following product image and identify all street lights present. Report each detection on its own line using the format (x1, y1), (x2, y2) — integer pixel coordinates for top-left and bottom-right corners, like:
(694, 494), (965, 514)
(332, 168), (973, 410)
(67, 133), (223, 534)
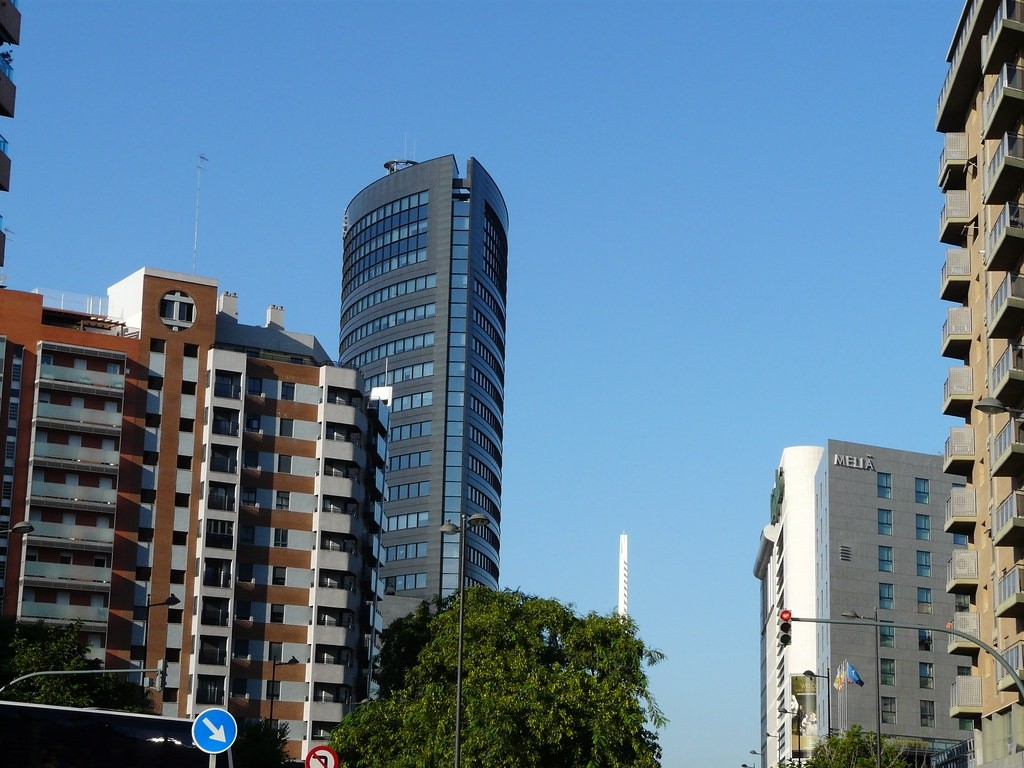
(143, 591), (180, 691)
(439, 512), (490, 768)
(268, 654), (299, 728)
(778, 704), (801, 768)
(841, 605), (893, 768)
(802, 668), (831, 737)
(750, 750), (766, 768)
(763, 732), (780, 768)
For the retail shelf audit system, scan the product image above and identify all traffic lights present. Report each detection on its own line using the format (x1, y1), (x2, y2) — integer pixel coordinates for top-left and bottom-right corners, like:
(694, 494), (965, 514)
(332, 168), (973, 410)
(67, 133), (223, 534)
(778, 608), (791, 647)
